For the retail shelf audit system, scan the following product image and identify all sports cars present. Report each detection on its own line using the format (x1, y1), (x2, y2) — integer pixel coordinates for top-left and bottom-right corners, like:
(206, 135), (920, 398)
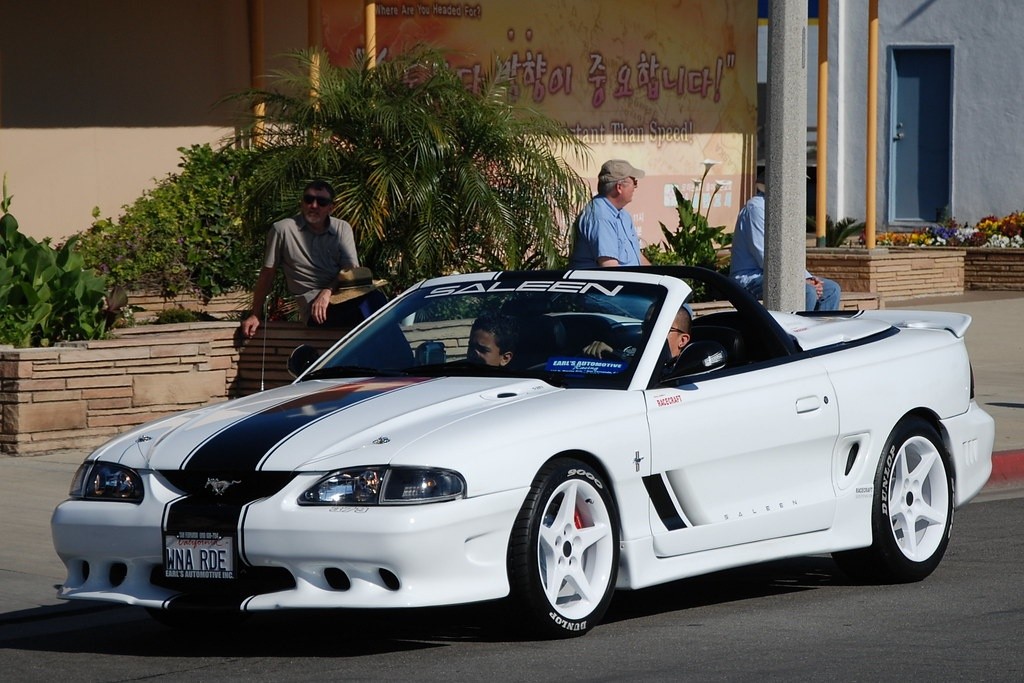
(50, 262), (996, 639)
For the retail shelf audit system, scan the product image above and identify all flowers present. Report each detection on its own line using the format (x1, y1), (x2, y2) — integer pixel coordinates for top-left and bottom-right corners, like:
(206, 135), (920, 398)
(857, 208), (1024, 251)
(637, 159), (735, 303)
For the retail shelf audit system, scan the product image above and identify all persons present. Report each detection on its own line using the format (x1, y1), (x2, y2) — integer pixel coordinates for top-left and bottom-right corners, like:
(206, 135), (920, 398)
(730, 171), (841, 311)
(583, 301), (692, 374)
(570, 160), (651, 267)
(241, 180), (415, 367)
(467, 312), (519, 366)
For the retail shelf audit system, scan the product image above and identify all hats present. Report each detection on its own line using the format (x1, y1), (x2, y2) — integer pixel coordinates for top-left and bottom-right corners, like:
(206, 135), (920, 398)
(598, 159), (645, 183)
(329, 266), (388, 304)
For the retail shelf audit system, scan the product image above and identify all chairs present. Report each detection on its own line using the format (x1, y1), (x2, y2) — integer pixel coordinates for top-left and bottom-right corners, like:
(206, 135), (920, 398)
(689, 325), (742, 369)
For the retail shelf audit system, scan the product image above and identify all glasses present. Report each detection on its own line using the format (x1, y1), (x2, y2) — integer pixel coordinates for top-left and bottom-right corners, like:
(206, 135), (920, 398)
(624, 179), (638, 189)
(302, 193), (334, 208)
(640, 320), (687, 336)
